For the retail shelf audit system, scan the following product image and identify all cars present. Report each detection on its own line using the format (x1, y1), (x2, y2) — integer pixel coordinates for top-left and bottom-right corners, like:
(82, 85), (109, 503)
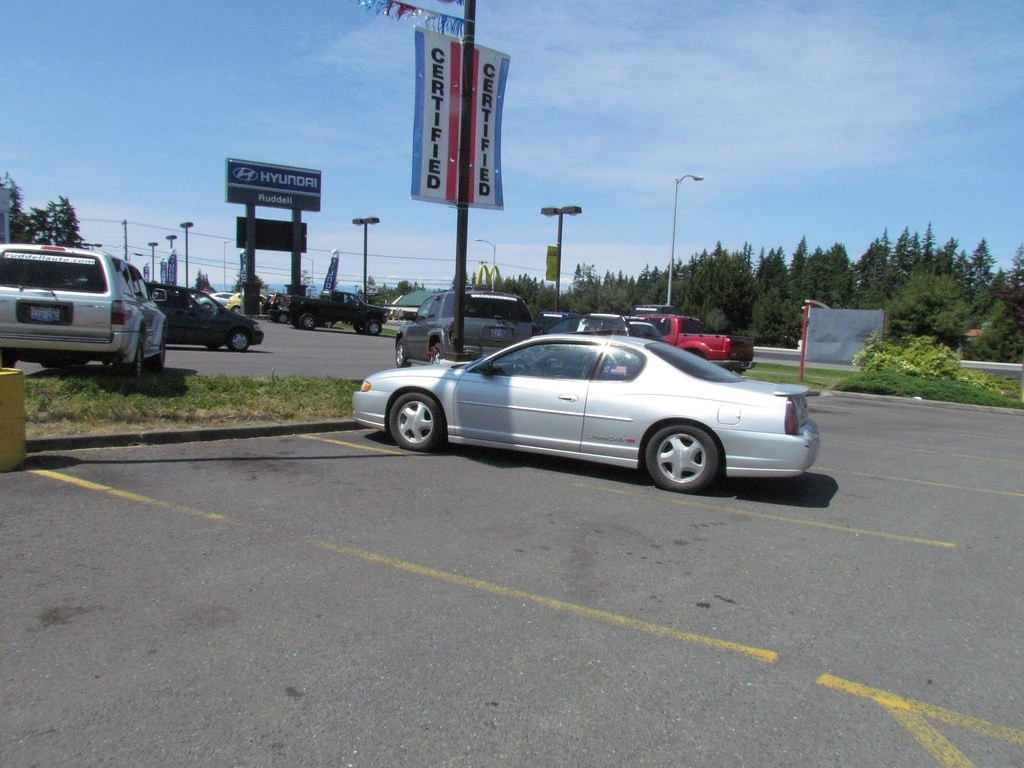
(353, 334), (820, 493)
(534, 305), (684, 342)
(145, 283), (291, 352)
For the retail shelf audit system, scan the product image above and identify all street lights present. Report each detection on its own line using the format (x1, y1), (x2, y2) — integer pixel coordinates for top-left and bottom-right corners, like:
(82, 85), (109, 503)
(352, 218), (380, 303)
(224, 241), (231, 292)
(165, 235), (178, 250)
(179, 221), (193, 288)
(667, 175), (703, 306)
(475, 239), (495, 292)
(148, 243), (159, 281)
(540, 206), (583, 315)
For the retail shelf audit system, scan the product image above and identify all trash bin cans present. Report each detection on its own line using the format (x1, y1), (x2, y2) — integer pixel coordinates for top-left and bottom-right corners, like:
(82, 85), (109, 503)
(0, 367), (26, 473)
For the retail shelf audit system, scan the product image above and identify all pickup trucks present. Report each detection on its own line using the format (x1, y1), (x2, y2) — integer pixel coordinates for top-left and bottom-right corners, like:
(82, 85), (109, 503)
(290, 291), (391, 337)
(638, 315), (754, 375)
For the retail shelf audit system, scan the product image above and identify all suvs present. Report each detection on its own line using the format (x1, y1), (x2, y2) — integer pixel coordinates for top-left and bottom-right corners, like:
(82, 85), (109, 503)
(395, 291), (544, 368)
(0, 245), (168, 377)
